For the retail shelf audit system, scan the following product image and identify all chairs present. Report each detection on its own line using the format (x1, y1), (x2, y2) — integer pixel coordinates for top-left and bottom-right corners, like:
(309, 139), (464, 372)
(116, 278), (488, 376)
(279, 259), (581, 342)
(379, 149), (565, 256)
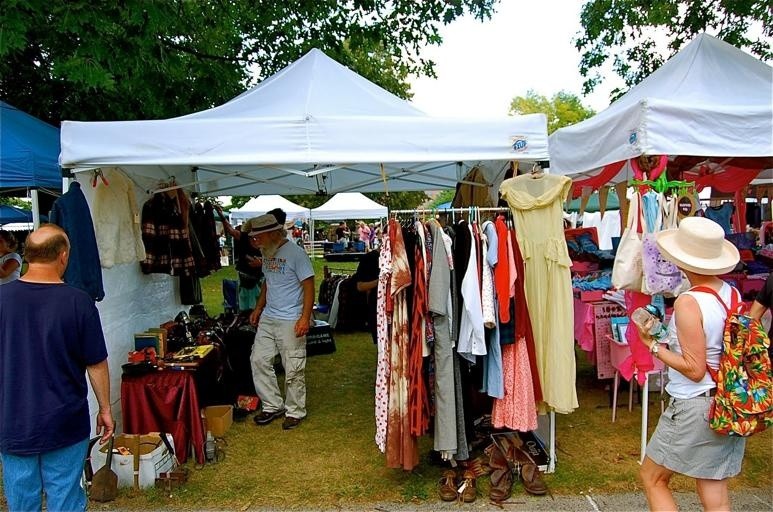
(304, 241), (335, 258)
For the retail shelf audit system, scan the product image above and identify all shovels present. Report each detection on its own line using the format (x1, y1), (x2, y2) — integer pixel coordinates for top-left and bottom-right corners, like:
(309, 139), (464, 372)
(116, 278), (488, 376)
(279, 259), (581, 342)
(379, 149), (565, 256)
(89, 419), (118, 503)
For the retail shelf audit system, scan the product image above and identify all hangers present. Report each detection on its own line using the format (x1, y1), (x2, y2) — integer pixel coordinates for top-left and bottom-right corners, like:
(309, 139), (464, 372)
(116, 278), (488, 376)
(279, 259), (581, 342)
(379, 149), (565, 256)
(453, 208), (455, 224)
(422, 206), (424, 222)
(475, 207), (476, 224)
(436, 207), (439, 221)
(478, 206), (482, 230)
(469, 207), (471, 224)
(444, 206), (447, 225)
(458, 207), (464, 224)
(432, 206), (435, 218)
(629, 169), (695, 194)
(395, 209), (397, 222)
(532, 163), (538, 177)
(472, 206), (473, 222)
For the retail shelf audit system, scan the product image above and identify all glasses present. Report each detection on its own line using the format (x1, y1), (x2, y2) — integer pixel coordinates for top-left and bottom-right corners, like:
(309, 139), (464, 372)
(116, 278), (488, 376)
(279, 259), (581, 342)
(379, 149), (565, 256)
(249, 235), (265, 241)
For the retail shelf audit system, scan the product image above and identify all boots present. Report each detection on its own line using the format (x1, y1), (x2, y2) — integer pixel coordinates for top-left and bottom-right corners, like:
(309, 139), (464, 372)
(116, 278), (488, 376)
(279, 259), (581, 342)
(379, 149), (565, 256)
(508, 445), (548, 495)
(483, 444), (513, 501)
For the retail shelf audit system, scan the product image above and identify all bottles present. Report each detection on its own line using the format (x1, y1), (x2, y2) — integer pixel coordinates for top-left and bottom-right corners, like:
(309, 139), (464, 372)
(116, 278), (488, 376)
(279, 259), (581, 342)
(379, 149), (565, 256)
(632, 307), (674, 347)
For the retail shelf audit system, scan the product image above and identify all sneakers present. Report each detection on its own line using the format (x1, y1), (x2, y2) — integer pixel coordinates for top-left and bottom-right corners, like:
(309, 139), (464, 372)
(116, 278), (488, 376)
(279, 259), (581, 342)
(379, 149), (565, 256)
(254, 409), (287, 424)
(282, 413), (305, 430)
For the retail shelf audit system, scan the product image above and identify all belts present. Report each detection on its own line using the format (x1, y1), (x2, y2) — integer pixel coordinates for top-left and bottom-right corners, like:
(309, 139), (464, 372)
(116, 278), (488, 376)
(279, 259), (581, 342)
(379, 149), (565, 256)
(698, 387), (716, 398)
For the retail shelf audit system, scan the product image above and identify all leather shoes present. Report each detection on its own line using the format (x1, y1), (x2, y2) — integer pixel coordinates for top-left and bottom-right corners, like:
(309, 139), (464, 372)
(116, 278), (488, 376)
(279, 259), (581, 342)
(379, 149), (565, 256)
(459, 469), (478, 504)
(438, 470), (457, 501)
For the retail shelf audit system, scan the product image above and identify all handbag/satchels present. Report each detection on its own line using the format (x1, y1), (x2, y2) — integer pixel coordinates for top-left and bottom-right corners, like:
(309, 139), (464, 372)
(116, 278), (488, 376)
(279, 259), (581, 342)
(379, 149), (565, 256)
(611, 191), (647, 291)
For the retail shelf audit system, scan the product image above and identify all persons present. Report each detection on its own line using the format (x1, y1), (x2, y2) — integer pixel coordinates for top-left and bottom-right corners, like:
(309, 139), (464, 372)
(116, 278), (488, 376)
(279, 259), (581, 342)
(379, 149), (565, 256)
(0, 228), (22, 283)
(0, 224), (113, 511)
(284, 218), (327, 244)
(354, 242), (381, 344)
(335, 220), (351, 248)
(746, 251), (773, 366)
(246, 213), (315, 430)
(211, 199), (263, 312)
(354, 220), (384, 253)
(219, 234), (227, 253)
(633, 216), (751, 510)
(266, 209), (286, 228)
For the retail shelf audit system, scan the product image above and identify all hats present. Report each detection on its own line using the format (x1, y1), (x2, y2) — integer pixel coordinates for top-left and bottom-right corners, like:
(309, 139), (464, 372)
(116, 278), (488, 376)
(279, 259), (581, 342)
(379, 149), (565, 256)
(654, 215), (741, 276)
(247, 213), (284, 237)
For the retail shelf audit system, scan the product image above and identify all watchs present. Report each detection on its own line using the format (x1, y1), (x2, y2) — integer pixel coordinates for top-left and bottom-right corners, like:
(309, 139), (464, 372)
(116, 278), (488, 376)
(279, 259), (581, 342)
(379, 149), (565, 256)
(651, 342), (660, 358)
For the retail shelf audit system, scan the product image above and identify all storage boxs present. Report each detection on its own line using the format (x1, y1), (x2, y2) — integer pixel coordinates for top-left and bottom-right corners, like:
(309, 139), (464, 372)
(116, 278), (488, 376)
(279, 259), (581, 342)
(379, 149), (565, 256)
(604, 335), (666, 371)
(90, 433), (177, 489)
(201, 406), (232, 437)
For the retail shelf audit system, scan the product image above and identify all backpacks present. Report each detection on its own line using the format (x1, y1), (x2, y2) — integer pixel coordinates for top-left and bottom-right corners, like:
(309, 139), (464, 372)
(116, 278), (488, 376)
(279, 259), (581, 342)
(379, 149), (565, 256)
(675, 282), (771, 436)
